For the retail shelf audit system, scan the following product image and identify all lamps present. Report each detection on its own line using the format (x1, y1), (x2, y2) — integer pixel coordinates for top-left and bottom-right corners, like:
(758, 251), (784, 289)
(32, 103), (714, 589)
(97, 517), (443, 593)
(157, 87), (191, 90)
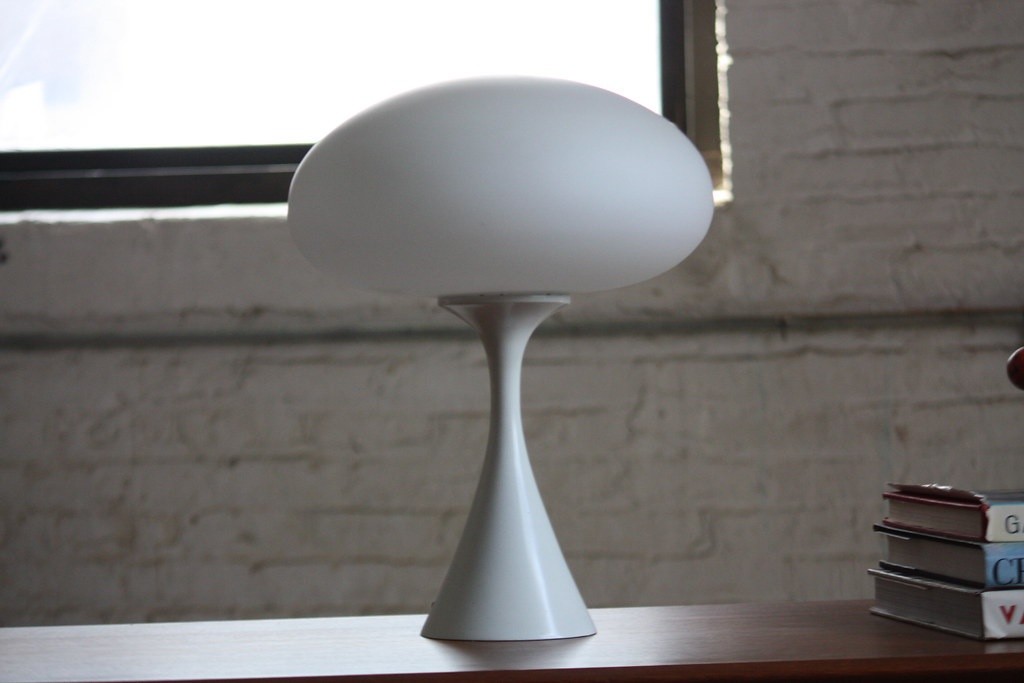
(288, 74), (715, 644)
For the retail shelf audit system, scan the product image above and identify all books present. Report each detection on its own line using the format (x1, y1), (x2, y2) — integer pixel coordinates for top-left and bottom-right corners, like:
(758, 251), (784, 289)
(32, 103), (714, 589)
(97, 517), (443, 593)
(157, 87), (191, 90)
(867, 479), (1024, 643)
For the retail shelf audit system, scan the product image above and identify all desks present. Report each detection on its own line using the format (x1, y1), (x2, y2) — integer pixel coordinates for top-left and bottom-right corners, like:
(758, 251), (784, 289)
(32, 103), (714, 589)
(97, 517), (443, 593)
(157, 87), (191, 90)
(0, 597), (1024, 683)
